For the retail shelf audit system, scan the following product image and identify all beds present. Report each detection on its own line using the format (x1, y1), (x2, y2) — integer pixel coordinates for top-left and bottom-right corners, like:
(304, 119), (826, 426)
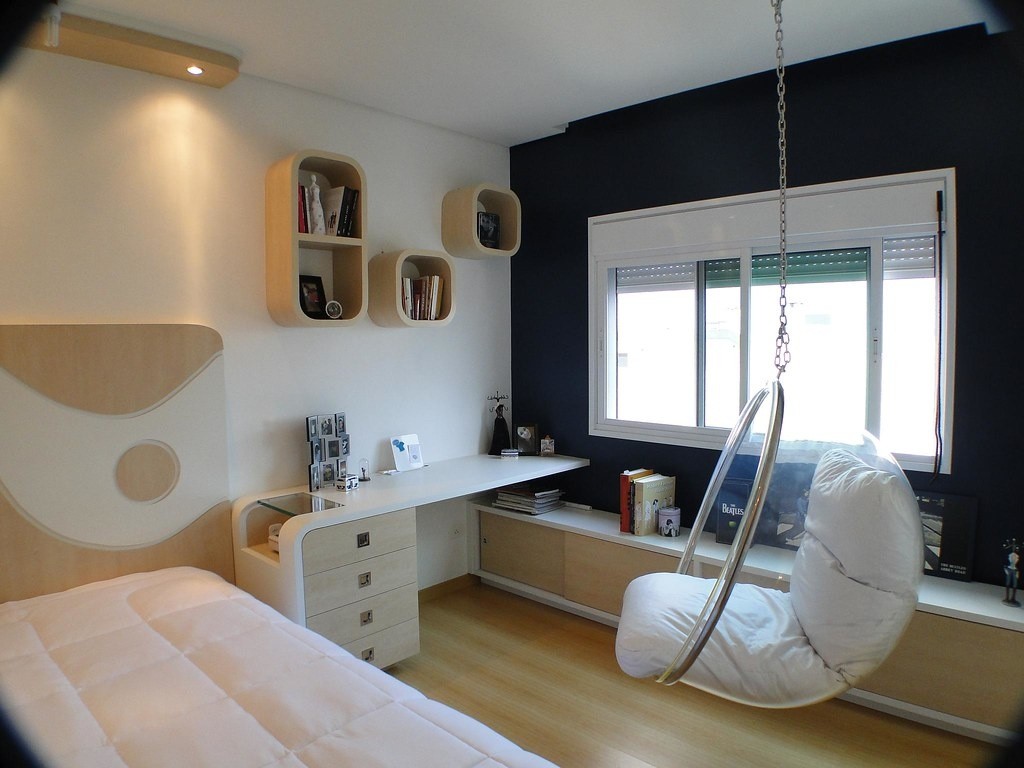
(0, 324), (560, 768)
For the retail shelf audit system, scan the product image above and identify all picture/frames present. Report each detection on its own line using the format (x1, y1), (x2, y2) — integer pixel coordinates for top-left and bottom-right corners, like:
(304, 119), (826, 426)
(306, 412), (351, 493)
(299, 275), (329, 320)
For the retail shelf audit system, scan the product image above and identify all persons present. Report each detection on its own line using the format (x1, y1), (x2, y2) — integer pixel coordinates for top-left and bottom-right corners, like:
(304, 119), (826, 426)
(785, 487), (811, 542)
(309, 174), (326, 236)
(1004, 553), (1019, 603)
(488, 403), (511, 456)
(517, 427), (535, 452)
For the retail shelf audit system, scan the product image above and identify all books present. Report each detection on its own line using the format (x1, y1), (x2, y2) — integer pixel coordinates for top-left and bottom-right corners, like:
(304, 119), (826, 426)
(716, 478), (757, 549)
(298, 181), (312, 234)
(619, 467), (676, 536)
(323, 185), (359, 237)
(491, 487), (566, 515)
(400, 275), (444, 321)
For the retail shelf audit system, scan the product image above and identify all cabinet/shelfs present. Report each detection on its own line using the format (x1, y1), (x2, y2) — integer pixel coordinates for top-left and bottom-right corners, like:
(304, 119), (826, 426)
(265, 148), (368, 328)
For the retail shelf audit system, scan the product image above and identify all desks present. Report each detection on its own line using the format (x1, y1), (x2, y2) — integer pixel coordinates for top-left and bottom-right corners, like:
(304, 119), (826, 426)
(234, 453), (592, 669)
